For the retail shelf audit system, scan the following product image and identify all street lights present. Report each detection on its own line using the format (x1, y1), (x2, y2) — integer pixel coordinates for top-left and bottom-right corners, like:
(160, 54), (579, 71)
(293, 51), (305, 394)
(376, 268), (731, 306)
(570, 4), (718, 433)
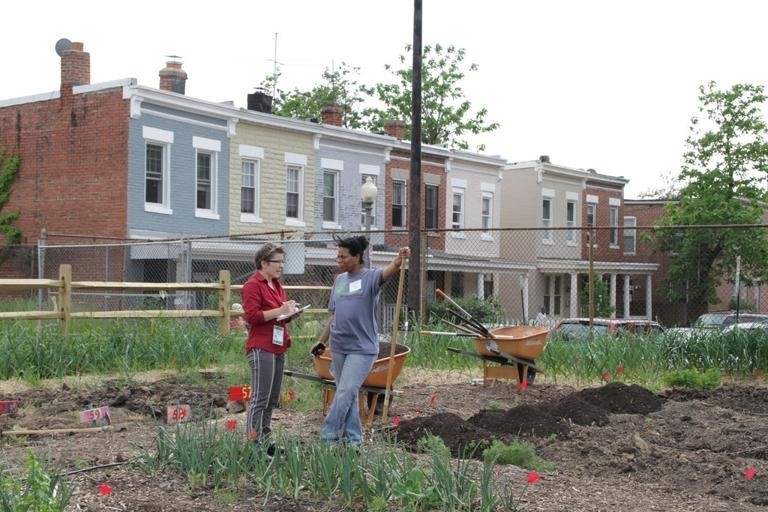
(358, 173), (379, 264)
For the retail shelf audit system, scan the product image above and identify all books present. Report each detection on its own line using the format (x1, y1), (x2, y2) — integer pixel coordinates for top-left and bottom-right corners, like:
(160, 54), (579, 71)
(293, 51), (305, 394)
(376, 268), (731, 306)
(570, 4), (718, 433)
(276, 304), (311, 322)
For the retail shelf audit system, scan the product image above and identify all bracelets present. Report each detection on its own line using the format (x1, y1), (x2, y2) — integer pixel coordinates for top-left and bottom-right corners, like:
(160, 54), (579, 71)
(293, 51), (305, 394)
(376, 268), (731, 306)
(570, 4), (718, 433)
(393, 258), (400, 268)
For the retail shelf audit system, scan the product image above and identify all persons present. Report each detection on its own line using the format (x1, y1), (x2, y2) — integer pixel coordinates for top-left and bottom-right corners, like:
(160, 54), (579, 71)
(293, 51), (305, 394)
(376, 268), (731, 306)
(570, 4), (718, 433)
(311, 234), (412, 458)
(241, 243), (303, 459)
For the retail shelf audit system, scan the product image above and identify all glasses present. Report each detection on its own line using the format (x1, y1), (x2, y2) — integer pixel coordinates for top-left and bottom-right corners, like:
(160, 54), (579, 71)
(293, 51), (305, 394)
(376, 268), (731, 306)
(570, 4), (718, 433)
(265, 259), (285, 264)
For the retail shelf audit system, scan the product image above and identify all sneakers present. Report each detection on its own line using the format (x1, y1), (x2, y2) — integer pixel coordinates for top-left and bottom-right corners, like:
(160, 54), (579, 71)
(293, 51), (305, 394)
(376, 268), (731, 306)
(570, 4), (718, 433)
(266, 445), (286, 457)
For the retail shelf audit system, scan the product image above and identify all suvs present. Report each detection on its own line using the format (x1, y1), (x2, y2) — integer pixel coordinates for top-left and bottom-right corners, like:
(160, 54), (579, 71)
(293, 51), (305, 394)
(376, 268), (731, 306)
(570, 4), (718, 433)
(553, 317), (667, 340)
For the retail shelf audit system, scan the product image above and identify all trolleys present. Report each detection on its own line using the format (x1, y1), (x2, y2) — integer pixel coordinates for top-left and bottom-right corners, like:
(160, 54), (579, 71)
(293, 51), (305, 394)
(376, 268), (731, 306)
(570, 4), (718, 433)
(317, 341), (412, 425)
(420, 325), (549, 388)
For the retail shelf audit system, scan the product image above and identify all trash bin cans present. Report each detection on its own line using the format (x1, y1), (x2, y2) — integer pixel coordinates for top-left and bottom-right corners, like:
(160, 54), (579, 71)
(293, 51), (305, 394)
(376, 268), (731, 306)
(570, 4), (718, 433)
(407, 306), (416, 319)
(281, 301), (301, 306)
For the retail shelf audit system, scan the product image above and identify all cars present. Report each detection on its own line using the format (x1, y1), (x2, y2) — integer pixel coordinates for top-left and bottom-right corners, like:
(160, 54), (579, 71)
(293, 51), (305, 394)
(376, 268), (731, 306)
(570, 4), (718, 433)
(663, 312), (768, 342)
